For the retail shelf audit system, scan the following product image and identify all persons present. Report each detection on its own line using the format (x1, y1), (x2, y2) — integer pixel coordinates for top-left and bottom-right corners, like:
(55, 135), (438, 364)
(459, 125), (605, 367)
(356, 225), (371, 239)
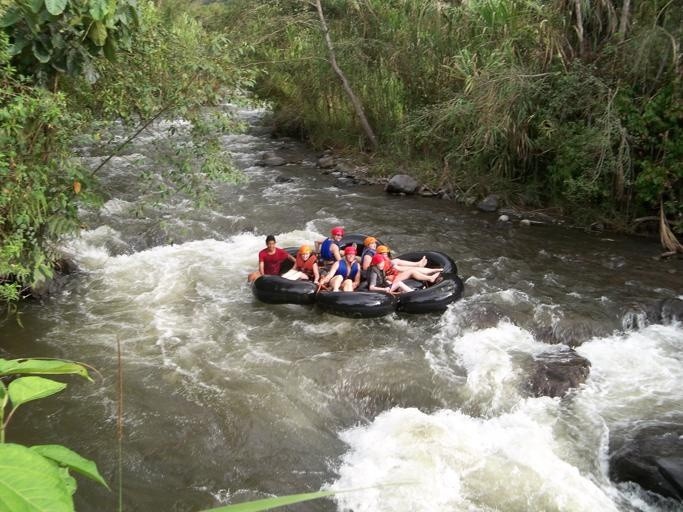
(361, 236), (444, 295)
(317, 227), (361, 267)
(248, 234), (296, 288)
(319, 247), (361, 292)
(281, 245), (320, 284)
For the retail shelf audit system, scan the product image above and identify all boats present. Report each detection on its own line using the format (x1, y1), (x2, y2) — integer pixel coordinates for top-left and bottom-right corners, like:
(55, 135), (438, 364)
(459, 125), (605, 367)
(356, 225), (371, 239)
(252, 233), (463, 319)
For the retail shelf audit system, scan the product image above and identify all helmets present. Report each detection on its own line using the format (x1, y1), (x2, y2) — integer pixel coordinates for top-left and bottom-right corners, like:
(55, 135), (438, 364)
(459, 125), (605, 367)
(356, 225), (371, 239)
(372, 254), (384, 264)
(299, 245), (311, 254)
(363, 236), (377, 246)
(377, 246), (389, 253)
(344, 247), (357, 255)
(331, 227), (344, 235)
